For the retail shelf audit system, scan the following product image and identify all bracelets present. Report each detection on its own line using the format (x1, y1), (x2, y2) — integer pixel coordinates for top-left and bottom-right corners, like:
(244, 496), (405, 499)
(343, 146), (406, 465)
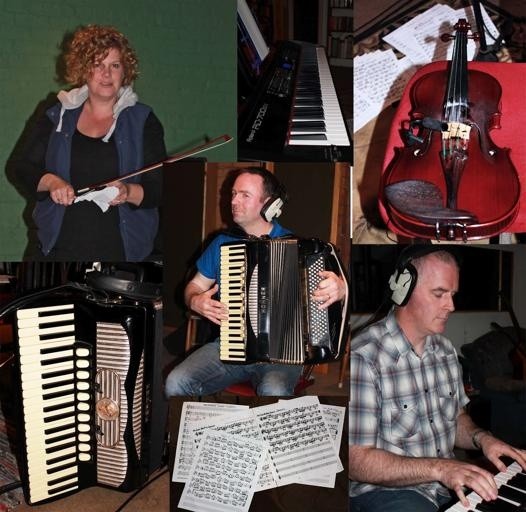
(470, 428), (494, 448)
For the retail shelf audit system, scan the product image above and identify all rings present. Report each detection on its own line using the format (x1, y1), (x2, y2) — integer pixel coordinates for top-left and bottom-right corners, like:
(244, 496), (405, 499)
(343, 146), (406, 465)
(327, 294), (331, 300)
(117, 199), (121, 204)
(58, 199), (63, 202)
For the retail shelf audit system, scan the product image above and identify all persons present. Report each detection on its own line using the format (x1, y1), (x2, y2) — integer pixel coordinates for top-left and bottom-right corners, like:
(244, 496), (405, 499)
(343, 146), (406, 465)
(349, 239), (526, 512)
(159, 165), (351, 401)
(5, 21), (175, 260)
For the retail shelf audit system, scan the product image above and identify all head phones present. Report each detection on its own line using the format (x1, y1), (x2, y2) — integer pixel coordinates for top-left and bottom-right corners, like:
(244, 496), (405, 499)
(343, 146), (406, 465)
(261, 183), (283, 223)
(387, 247), (442, 305)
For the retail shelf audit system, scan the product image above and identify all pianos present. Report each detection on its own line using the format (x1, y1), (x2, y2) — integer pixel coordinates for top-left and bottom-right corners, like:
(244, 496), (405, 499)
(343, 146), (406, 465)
(238, 41), (352, 163)
(438, 460), (526, 510)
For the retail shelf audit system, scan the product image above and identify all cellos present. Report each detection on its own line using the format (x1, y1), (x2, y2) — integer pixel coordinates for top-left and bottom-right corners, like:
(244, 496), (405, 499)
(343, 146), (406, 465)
(379, 19), (522, 242)
